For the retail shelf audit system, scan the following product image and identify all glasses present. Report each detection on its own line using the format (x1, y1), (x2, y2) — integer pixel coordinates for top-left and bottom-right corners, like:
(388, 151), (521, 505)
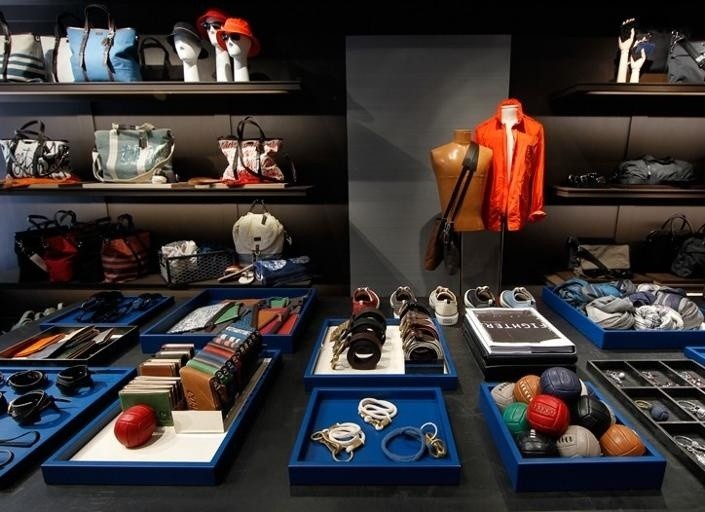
(201, 21), (224, 30)
(221, 32), (240, 41)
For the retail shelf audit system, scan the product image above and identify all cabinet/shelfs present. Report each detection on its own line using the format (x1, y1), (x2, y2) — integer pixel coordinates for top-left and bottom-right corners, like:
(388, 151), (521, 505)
(497, 0), (705, 298)
(0, 0), (351, 294)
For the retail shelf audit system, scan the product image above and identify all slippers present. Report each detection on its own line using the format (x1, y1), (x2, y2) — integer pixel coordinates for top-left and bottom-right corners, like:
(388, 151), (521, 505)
(217, 263), (254, 282)
(238, 271), (255, 285)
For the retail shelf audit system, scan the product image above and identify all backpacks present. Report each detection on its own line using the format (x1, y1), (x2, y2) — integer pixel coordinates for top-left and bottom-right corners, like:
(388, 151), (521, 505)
(217, 117), (285, 182)
(666, 29), (704, 84)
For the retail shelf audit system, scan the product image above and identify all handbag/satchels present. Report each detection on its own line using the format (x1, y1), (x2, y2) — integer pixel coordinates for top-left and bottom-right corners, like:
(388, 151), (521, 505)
(232, 199), (286, 261)
(100, 213), (153, 285)
(0, 14), (48, 85)
(91, 122), (177, 184)
(614, 154), (697, 185)
(423, 217), (461, 276)
(66, 4), (142, 83)
(564, 233), (633, 281)
(642, 213), (705, 278)
(0, 120), (73, 180)
(14, 209), (91, 286)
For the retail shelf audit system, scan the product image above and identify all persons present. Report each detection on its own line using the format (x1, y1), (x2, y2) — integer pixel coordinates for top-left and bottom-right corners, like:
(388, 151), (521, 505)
(430, 130), (492, 232)
(629, 37), (649, 83)
(164, 23), (209, 82)
(474, 98), (545, 231)
(215, 17), (262, 83)
(615, 17), (635, 84)
(196, 7), (233, 82)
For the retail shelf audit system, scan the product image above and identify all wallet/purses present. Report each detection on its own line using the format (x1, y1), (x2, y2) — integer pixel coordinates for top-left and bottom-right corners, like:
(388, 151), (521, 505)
(179, 323), (265, 411)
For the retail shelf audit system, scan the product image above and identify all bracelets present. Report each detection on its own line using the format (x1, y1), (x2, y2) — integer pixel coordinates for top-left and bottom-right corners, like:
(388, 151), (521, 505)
(310, 396), (450, 466)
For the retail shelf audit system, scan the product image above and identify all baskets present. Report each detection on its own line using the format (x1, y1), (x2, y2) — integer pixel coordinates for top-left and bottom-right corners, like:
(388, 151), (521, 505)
(157, 244), (233, 286)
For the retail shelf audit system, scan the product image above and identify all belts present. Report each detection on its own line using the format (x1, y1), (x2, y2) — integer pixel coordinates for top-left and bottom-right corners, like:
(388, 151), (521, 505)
(330, 308), (444, 370)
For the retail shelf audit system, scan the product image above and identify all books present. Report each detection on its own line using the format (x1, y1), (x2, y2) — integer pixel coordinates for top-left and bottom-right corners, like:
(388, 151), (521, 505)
(461, 305), (580, 384)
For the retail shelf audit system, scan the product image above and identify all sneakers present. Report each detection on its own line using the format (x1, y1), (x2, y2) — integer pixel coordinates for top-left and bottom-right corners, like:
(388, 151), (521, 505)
(499, 286), (538, 311)
(428, 285), (460, 325)
(351, 286), (381, 318)
(463, 285), (496, 308)
(389, 285), (418, 319)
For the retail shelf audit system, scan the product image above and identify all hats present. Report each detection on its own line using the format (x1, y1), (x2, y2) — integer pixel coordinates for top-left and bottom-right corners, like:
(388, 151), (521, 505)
(216, 16), (262, 57)
(165, 21), (208, 60)
(195, 8), (232, 42)
